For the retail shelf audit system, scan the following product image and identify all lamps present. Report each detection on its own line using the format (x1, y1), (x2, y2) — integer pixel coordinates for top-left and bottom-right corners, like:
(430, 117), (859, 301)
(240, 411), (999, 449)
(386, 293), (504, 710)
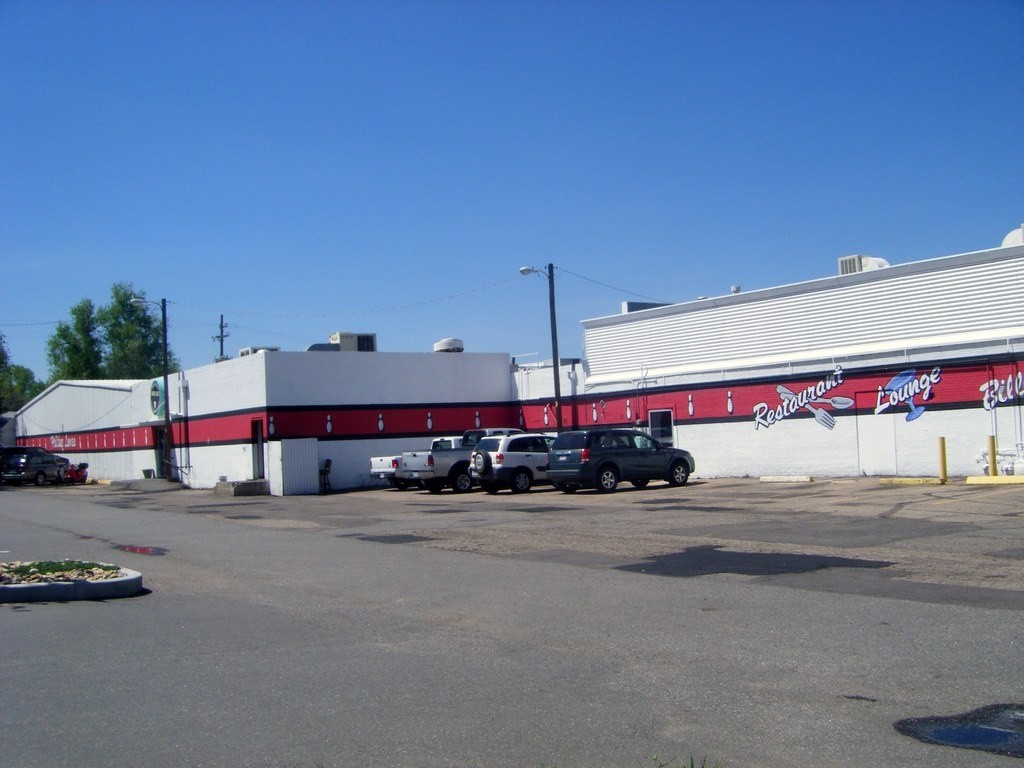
(834, 365), (843, 375)
(600, 400), (606, 408)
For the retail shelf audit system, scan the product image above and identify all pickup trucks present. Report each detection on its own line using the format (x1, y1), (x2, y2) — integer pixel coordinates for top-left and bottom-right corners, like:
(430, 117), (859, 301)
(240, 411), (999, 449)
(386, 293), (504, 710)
(370, 435), (476, 491)
(391, 429), (541, 495)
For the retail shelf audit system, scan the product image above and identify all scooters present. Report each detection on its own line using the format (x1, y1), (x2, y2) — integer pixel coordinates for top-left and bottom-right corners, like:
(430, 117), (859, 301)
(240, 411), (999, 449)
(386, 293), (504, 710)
(63, 462), (89, 486)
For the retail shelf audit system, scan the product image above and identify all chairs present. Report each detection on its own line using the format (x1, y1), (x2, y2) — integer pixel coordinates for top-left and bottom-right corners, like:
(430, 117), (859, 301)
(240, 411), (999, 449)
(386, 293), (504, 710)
(319, 459), (332, 491)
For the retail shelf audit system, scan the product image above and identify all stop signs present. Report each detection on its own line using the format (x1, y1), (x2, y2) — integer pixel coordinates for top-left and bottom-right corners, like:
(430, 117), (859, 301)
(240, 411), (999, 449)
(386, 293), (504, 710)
(150, 379), (164, 415)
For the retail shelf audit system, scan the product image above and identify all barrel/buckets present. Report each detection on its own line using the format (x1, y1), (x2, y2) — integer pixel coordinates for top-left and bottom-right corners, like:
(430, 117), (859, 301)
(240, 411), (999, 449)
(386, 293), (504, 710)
(142, 469), (153, 479)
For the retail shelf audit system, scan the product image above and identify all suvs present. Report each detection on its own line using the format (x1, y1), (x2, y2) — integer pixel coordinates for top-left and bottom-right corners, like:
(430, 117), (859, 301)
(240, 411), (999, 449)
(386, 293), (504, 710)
(0, 446), (69, 487)
(545, 429), (696, 494)
(467, 433), (557, 494)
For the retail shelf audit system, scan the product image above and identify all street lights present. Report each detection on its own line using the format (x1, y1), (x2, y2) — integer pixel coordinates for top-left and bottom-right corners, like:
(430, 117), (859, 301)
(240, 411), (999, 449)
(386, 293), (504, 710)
(131, 297), (173, 483)
(520, 262), (563, 431)
